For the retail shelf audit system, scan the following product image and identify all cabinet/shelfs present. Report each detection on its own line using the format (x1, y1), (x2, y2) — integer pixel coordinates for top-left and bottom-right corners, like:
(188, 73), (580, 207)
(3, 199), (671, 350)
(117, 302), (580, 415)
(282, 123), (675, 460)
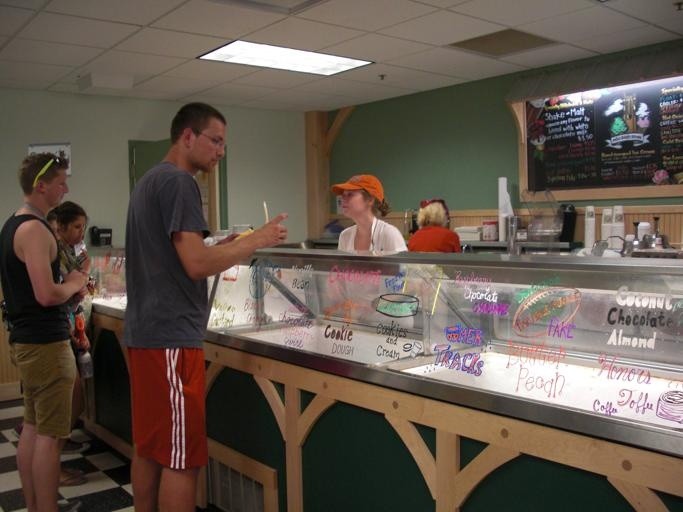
(76, 238), (683, 512)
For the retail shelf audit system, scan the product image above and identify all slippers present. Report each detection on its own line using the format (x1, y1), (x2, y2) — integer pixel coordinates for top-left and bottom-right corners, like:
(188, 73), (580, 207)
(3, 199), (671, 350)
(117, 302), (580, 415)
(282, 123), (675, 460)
(58, 472), (88, 485)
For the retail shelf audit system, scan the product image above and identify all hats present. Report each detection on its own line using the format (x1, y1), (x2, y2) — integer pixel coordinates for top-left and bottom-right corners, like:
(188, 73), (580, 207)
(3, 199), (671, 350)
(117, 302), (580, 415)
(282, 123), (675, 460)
(332, 174), (384, 203)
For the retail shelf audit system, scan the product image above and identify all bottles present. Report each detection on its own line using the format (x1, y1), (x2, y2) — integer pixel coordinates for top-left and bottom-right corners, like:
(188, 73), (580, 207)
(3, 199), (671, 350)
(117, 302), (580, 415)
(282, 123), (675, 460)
(399, 207), (418, 240)
(585, 205), (625, 249)
(78, 348), (95, 379)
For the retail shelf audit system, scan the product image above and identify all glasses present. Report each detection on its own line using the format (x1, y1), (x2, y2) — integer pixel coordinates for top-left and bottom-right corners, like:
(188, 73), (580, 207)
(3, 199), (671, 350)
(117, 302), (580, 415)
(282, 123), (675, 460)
(32, 157), (65, 187)
(198, 132), (225, 145)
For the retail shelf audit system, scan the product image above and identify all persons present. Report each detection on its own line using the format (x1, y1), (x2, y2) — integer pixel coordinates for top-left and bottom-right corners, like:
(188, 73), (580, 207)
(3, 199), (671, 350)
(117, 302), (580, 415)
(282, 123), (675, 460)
(325, 174), (414, 327)
(403, 197), (461, 339)
(119, 98), (288, 512)
(0, 150), (96, 512)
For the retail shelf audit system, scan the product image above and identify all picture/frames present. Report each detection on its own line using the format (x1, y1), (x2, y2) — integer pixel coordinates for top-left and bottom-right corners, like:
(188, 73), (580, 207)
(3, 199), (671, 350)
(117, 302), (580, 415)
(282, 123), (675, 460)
(124, 139), (227, 230)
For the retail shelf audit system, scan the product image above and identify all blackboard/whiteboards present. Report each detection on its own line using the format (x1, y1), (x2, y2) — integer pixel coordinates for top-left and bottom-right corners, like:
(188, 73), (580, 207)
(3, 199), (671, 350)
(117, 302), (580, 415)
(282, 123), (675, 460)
(517, 76), (683, 202)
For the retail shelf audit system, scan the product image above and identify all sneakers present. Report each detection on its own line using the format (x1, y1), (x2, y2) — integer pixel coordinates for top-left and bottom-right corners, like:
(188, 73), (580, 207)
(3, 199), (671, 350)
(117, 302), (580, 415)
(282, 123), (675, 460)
(63, 438), (90, 454)
(59, 499), (82, 511)
